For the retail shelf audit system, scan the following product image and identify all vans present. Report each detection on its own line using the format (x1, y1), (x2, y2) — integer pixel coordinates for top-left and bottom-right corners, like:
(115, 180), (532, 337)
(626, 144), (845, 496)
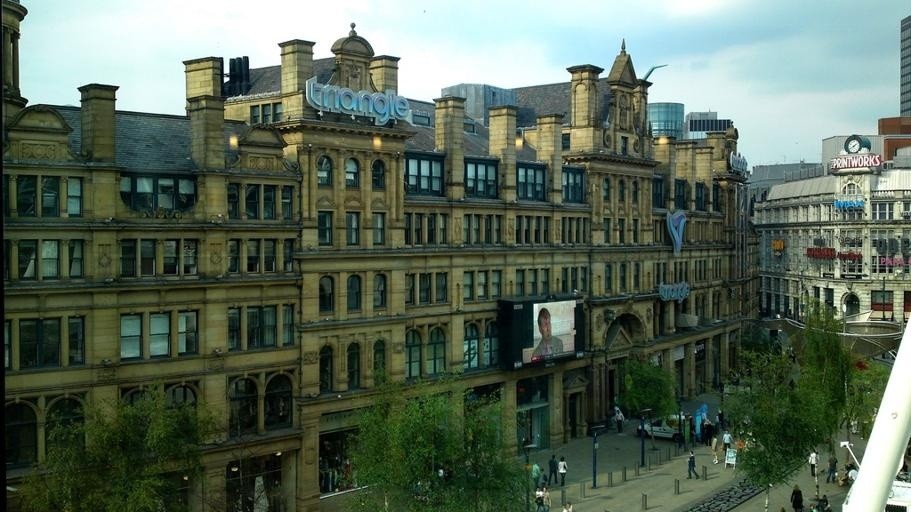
(638, 414), (685, 443)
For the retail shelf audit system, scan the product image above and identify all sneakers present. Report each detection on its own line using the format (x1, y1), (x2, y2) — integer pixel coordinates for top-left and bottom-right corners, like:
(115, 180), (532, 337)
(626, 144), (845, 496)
(686, 476), (700, 480)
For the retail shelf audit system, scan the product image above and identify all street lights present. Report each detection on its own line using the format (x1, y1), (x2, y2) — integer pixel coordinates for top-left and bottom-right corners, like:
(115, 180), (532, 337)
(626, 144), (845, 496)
(522, 434), (540, 511)
(640, 408), (650, 467)
(718, 382), (725, 430)
(589, 425), (606, 489)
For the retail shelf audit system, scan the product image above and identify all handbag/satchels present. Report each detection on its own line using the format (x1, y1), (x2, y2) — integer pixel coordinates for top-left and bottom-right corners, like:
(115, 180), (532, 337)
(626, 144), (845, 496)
(563, 463), (566, 470)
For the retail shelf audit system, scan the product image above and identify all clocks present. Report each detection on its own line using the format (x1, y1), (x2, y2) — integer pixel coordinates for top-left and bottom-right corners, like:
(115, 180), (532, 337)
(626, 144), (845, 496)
(848, 139), (860, 152)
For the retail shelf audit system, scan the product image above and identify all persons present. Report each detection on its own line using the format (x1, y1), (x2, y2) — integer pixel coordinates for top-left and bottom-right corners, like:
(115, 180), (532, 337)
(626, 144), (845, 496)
(612, 403), (620, 423)
(614, 410), (625, 433)
(614, 393), (620, 405)
(779, 461), (858, 512)
(808, 448), (817, 478)
(701, 408), (745, 465)
(530, 307), (564, 358)
(901, 461), (910, 473)
(530, 453), (576, 512)
(685, 450), (701, 480)
(839, 408), (879, 440)
(823, 451), (838, 485)
